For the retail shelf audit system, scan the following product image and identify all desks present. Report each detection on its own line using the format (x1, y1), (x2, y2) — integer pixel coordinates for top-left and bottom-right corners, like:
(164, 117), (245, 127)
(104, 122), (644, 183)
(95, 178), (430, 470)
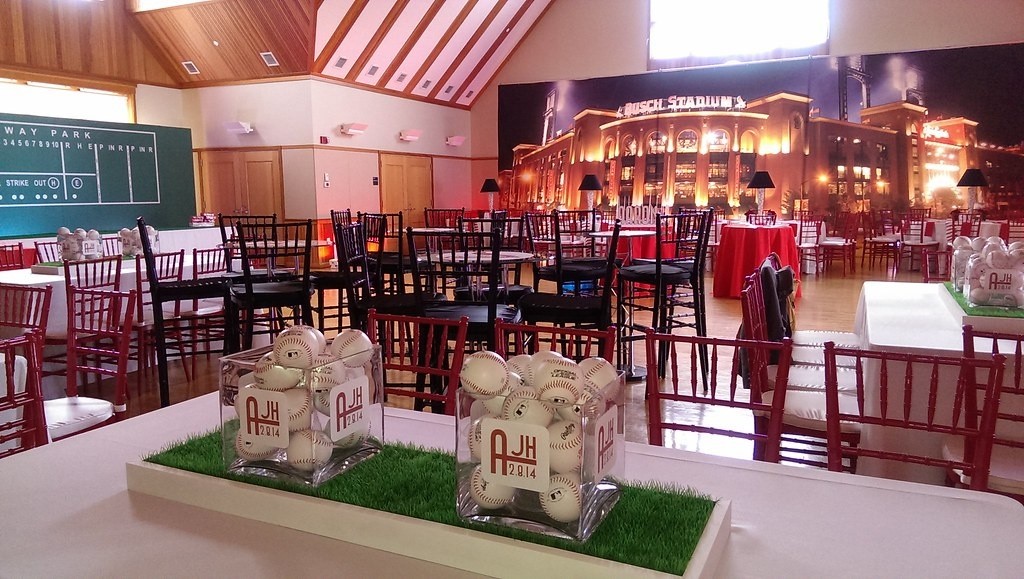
(0, 389), (1024, 579)
(396, 227), (467, 253)
(855, 281), (1024, 487)
(713, 224), (801, 298)
(410, 251), (534, 290)
(589, 230), (664, 266)
(222, 240), (335, 330)
(0, 251), (270, 389)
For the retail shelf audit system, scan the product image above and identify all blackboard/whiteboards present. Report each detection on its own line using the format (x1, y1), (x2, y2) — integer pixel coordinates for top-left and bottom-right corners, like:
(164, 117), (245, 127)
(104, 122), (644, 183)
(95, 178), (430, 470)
(0, 112), (197, 241)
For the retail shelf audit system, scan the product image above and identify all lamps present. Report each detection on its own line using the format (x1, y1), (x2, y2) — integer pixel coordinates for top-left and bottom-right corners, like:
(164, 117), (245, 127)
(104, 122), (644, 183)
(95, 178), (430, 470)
(956, 169), (989, 220)
(578, 174), (603, 210)
(479, 179), (501, 211)
(747, 171), (776, 224)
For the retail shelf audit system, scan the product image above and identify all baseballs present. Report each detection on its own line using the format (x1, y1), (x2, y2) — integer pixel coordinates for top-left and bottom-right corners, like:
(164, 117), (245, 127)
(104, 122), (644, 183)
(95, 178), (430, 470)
(236, 325), (374, 472)
(459, 350), (621, 524)
(121, 225), (159, 256)
(951, 236), (1024, 306)
(58, 226), (103, 261)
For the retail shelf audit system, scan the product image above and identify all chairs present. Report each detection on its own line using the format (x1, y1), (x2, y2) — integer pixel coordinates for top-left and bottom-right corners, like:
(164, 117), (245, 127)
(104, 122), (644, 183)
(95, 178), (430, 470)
(0, 204), (1024, 492)
